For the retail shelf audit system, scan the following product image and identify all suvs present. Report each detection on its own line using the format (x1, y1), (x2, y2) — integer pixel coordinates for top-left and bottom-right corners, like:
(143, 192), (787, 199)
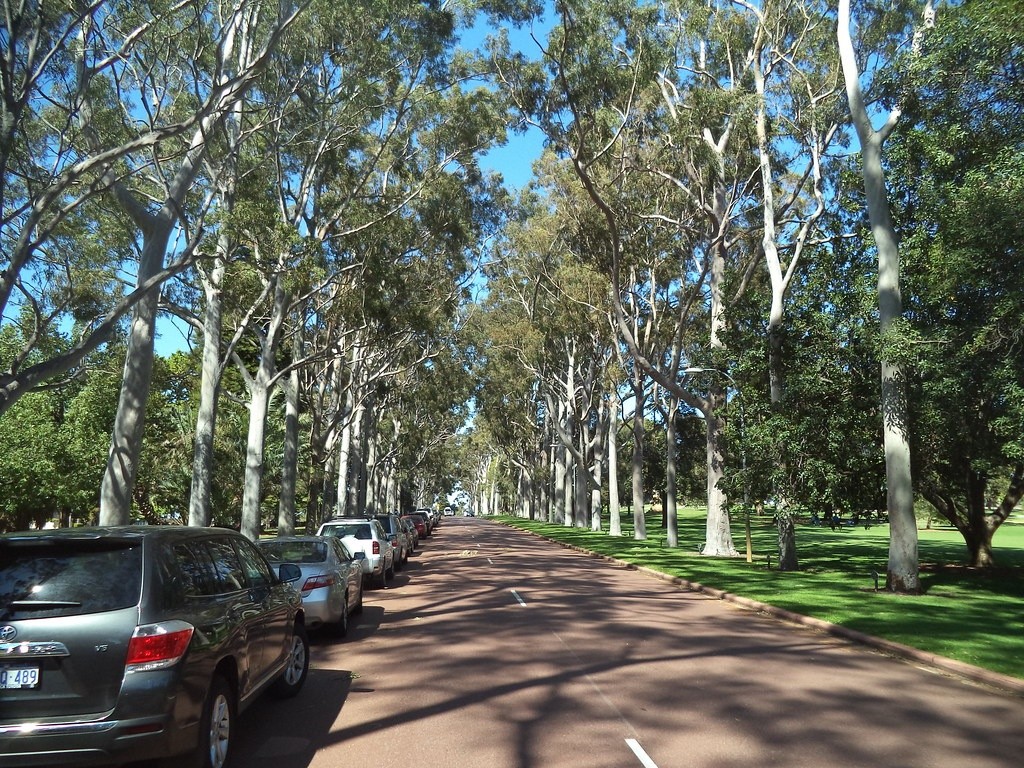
(315, 516), (394, 585)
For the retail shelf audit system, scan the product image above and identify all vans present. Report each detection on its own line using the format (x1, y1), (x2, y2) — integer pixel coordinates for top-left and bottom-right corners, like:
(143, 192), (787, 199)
(0, 524), (309, 768)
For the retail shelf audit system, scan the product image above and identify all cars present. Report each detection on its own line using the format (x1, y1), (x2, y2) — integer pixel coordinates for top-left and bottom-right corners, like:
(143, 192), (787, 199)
(373, 507), (454, 569)
(253, 536), (364, 631)
